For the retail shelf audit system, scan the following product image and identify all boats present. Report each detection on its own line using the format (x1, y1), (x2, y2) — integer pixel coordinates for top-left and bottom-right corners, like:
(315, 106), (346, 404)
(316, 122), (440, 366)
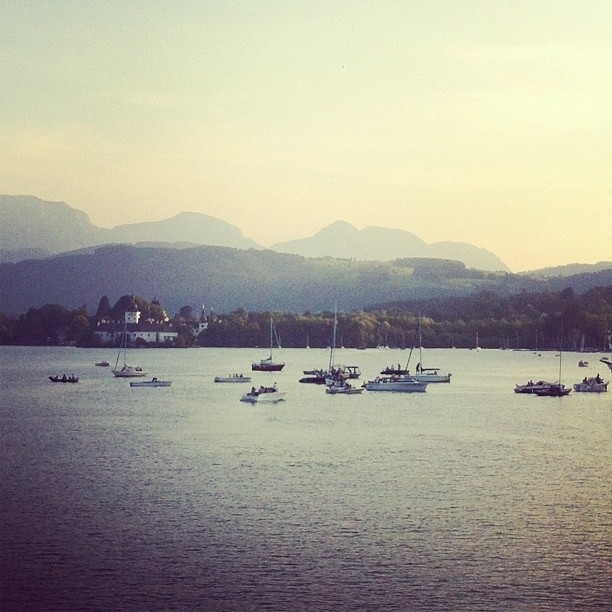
(129, 380), (172, 387)
(213, 374), (251, 382)
(531, 335), (573, 396)
(325, 385), (364, 396)
(302, 297), (362, 379)
(573, 374), (611, 393)
(251, 318), (286, 372)
(514, 381), (567, 393)
(413, 311), (452, 383)
(299, 375), (348, 385)
(578, 359), (589, 368)
(47, 374), (79, 383)
(238, 388), (287, 404)
(379, 319), (420, 375)
(94, 360), (109, 366)
(111, 311), (147, 378)
(361, 376), (428, 394)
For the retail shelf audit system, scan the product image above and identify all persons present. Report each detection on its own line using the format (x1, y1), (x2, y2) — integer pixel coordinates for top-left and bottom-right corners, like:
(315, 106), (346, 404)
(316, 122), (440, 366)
(596, 373), (602, 384)
(583, 377), (588, 384)
(54, 375), (59, 380)
(62, 374), (67, 380)
(250, 387), (256, 395)
(527, 380), (533, 386)
(313, 367), (342, 377)
(151, 377), (157, 382)
(416, 362), (421, 374)
(387, 363), (401, 370)
(391, 372), (410, 382)
